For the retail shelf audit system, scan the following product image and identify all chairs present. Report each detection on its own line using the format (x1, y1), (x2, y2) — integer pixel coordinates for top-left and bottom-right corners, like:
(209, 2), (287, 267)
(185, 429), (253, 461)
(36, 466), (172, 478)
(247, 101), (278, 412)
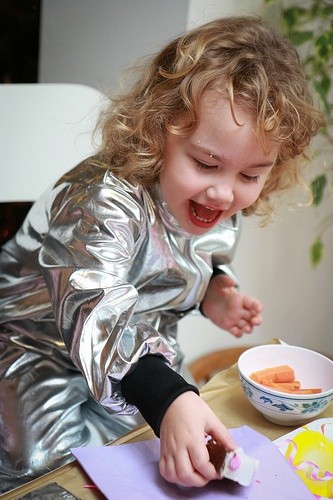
(0, 84), (116, 202)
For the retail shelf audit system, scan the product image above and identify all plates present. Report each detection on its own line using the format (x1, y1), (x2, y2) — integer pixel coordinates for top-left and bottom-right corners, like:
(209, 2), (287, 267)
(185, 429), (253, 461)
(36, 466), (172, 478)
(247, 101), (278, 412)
(70, 425), (317, 500)
(271, 417), (333, 500)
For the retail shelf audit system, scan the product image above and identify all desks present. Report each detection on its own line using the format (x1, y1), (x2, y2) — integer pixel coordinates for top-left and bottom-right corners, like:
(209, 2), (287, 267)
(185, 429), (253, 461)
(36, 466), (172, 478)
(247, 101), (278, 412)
(1, 349), (333, 500)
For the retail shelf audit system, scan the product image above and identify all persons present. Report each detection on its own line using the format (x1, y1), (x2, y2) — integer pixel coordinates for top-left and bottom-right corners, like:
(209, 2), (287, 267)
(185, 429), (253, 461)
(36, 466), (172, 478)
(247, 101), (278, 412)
(0, 17), (327, 495)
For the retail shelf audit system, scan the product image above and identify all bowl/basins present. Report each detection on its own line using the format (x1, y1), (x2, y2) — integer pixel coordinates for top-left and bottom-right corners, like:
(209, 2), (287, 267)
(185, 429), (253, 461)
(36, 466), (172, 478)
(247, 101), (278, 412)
(237, 344), (333, 427)
